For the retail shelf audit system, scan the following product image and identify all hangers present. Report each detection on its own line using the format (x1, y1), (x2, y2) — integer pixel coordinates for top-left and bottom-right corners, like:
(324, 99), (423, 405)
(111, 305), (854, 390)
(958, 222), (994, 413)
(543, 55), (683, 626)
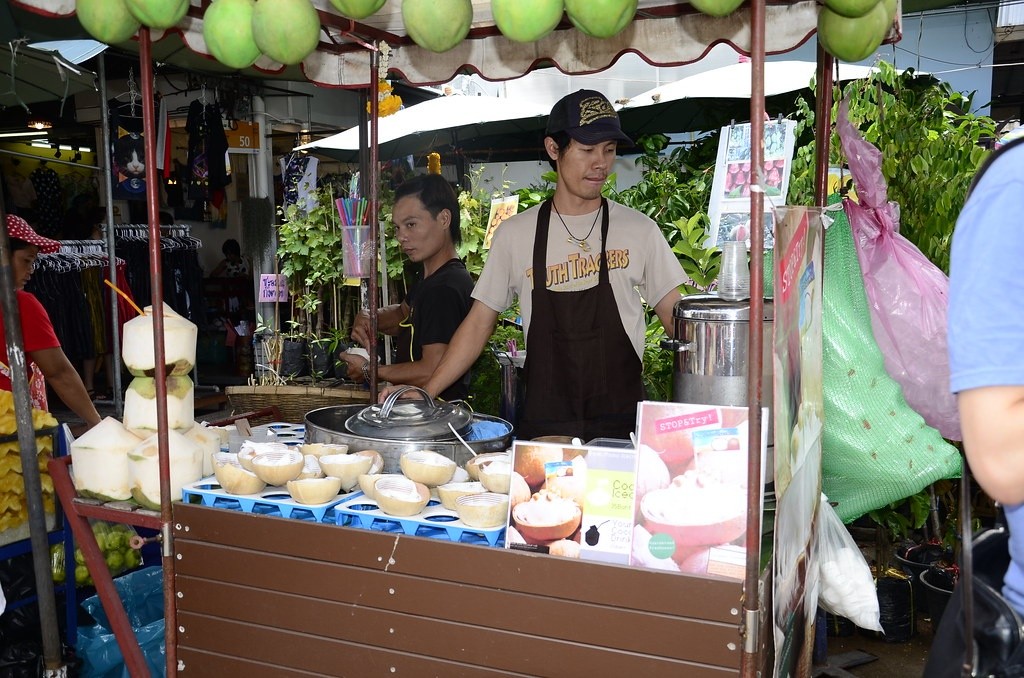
(288, 141), (307, 155)
(114, 79), (158, 108)
(31, 224), (202, 273)
(197, 84), (210, 106)
(108, 91), (144, 118)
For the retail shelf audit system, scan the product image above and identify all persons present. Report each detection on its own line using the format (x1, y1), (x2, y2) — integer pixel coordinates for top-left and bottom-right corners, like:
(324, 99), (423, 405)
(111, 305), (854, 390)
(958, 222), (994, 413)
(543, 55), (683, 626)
(209, 239), (256, 289)
(0, 214), (104, 445)
(949, 107), (1024, 678)
(338, 174), (481, 407)
(75, 206), (120, 401)
(157, 211), (205, 288)
(378, 89), (699, 449)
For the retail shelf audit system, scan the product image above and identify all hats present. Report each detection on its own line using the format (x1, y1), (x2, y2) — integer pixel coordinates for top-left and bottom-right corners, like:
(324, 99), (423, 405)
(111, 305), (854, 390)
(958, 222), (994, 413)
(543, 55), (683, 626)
(545, 89), (635, 150)
(5, 213), (62, 254)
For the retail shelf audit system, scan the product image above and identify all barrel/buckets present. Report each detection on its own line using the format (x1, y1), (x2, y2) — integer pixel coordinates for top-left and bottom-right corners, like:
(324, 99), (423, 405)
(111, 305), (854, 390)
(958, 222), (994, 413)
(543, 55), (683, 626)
(894, 544), (956, 632)
(660, 294), (775, 448)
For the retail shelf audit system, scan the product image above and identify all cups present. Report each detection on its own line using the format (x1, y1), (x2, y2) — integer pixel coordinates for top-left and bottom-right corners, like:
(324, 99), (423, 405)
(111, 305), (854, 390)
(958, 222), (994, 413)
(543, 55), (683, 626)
(718, 241), (751, 302)
(340, 225), (370, 279)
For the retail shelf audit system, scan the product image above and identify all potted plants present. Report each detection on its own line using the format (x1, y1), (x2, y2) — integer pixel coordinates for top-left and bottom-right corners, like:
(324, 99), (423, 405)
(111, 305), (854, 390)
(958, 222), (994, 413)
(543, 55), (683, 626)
(280, 202), (404, 383)
(779, 65), (999, 637)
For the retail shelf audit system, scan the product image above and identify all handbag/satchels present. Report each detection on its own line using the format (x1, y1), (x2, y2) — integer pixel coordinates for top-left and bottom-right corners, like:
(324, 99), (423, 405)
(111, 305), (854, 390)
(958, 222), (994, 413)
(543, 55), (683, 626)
(923, 525), (1024, 678)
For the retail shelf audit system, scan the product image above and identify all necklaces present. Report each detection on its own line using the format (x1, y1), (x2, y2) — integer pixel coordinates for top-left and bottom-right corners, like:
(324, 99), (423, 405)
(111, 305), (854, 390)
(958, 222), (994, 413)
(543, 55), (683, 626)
(553, 194), (604, 252)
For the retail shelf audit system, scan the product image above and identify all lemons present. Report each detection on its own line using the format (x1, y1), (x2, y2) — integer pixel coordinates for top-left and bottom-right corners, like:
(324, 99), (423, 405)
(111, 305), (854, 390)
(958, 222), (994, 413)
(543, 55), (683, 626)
(47, 522), (142, 586)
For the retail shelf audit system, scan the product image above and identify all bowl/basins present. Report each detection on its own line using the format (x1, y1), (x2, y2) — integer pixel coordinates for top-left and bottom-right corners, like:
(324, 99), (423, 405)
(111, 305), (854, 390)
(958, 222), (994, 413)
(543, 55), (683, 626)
(498, 351), (527, 368)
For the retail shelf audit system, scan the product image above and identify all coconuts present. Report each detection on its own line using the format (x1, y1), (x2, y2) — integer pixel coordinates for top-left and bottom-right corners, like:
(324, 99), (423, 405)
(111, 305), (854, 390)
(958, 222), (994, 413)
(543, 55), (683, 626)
(67, 301), (219, 511)
(73, 0), (901, 70)
(509, 444), (588, 558)
(633, 400), (747, 575)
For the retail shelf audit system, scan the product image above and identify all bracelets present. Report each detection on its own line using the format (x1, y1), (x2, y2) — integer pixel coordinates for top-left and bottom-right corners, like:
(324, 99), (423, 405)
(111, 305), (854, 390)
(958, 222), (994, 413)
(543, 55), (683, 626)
(362, 361), (374, 385)
